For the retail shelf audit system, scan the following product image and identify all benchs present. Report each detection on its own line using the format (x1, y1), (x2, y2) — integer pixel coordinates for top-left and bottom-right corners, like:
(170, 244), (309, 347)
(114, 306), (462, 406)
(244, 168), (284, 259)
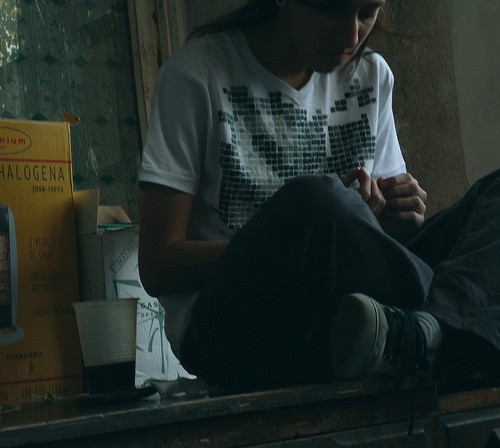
(0, 377), (500, 448)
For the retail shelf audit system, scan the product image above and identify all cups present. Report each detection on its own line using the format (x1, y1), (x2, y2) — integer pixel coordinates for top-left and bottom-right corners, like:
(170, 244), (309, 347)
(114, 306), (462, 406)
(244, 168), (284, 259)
(72, 298), (140, 395)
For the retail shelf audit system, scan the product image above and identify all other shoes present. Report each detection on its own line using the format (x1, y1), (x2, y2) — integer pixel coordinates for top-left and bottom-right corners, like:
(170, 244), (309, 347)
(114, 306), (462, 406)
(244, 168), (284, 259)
(324, 292), (443, 385)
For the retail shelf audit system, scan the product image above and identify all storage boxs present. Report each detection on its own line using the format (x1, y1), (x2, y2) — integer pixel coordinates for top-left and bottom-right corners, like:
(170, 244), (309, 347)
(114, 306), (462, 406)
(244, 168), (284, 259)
(71, 186), (190, 389)
(0, 119), (84, 408)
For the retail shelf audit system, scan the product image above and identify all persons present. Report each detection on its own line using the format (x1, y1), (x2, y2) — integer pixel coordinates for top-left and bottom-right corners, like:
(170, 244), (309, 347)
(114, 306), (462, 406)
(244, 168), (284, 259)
(138, 0), (499, 383)
